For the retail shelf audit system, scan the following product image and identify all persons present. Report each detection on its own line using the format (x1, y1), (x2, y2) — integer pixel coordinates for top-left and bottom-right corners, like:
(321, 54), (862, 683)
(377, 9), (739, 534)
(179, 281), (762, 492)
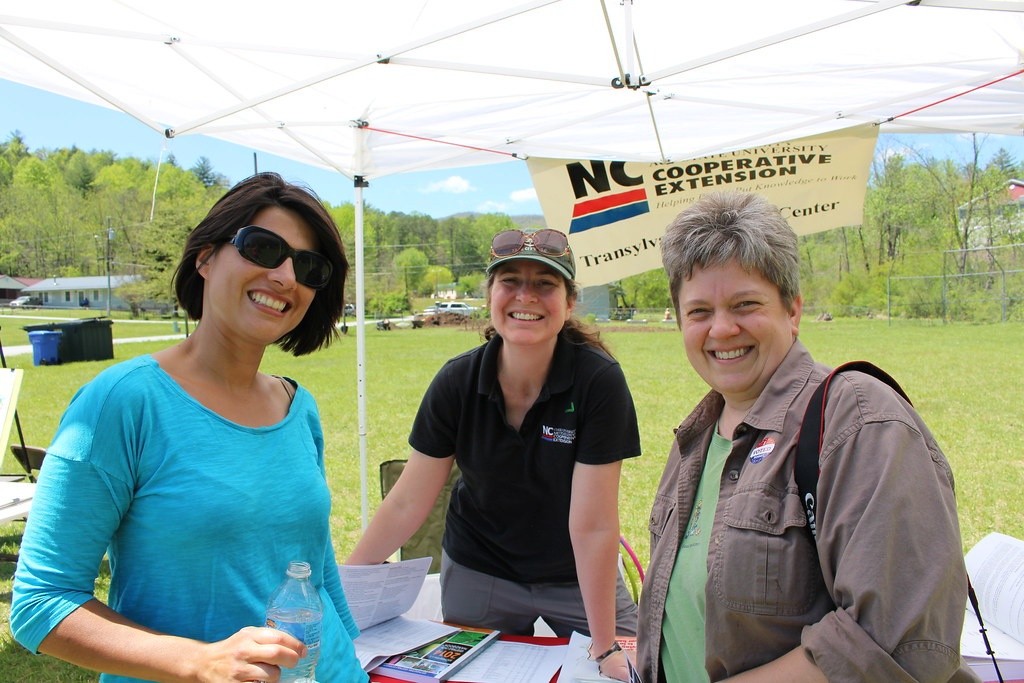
(664, 308), (672, 320)
(411, 309), (423, 328)
(635, 192), (982, 683)
(10, 171), (376, 683)
(340, 227), (638, 683)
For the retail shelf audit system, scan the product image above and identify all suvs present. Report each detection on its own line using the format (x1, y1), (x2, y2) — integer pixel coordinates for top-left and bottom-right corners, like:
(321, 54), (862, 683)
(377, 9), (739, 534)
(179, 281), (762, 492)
(422, 301), (479, 319)
(344, 303), (367, 316)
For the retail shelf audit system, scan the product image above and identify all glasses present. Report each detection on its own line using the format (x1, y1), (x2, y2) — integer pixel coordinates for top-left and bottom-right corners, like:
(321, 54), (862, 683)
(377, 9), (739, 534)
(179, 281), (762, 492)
(486, 228), (572, 270)
(227, 225), (333, 293)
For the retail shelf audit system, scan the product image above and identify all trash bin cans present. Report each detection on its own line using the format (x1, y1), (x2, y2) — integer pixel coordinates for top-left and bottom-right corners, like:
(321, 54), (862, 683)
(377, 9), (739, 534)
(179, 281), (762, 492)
(25, 330), (63, 366)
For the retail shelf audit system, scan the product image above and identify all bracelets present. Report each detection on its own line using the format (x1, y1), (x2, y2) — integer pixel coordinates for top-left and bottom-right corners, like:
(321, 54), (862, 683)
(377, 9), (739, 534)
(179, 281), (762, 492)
(595, 637), (621, 665)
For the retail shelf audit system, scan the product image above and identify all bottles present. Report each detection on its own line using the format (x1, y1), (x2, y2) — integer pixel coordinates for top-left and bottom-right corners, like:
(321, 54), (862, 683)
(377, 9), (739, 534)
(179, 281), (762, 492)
(264, 561), (323, 683)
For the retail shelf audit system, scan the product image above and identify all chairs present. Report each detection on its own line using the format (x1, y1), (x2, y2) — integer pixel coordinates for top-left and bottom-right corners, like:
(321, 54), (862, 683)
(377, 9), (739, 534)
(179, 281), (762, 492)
(380, 461), (463, 576)
(12, 445), (46, 484)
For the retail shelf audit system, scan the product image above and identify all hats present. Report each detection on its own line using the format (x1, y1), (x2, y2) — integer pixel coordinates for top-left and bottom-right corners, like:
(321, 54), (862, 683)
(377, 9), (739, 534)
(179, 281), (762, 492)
(487, 227), (577, 281)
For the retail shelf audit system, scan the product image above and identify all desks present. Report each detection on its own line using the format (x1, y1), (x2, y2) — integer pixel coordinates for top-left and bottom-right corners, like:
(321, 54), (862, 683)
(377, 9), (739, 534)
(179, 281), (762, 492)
(368, 632), (571, 683)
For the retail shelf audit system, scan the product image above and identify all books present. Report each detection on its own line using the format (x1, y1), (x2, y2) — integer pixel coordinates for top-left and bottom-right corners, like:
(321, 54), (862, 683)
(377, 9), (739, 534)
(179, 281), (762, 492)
(955, 531), (1024, 661)
(554, 630), (639, 683)
(368, 626), (501, 683)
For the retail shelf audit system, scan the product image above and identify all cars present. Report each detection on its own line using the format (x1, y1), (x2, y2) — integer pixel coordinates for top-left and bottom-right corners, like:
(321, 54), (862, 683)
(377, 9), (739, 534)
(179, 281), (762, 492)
(9, 296), (43, 309)
